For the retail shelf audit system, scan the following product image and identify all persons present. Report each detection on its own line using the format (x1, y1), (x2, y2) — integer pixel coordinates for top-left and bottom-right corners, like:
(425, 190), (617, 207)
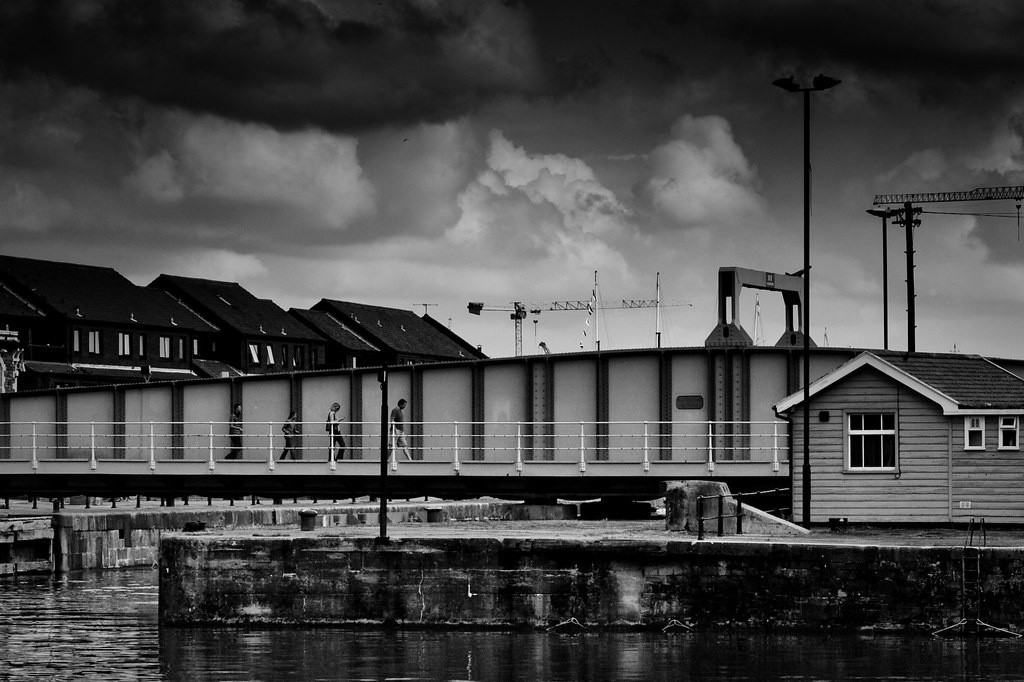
(387, 399), (412, 460)
(224, 403), (242, 459)
(326, 403), (345, 459)
(279, 412), (299, 459)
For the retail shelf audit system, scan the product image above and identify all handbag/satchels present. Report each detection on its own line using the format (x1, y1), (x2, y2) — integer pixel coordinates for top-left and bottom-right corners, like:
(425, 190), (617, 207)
(326, 412), (339, 433)
